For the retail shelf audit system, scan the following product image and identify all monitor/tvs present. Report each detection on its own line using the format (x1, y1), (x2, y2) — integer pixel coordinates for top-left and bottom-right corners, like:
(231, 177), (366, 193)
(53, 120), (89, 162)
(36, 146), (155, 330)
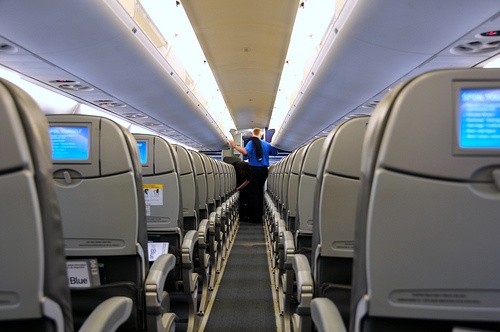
(48, 123), (91, 163)
(452, 81), (500, 155)
(136, 140), (149, 167)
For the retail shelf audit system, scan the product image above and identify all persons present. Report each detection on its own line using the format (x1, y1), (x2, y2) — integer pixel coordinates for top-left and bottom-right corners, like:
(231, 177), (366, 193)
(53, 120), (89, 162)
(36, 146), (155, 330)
(234, 161), (251, 190)
(230, 128), (279, 223)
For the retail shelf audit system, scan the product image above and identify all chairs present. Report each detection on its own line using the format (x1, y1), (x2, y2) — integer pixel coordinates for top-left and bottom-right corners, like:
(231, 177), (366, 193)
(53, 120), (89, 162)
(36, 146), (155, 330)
(0, 77), (240, 332)
(265, 67), (500, 332)
